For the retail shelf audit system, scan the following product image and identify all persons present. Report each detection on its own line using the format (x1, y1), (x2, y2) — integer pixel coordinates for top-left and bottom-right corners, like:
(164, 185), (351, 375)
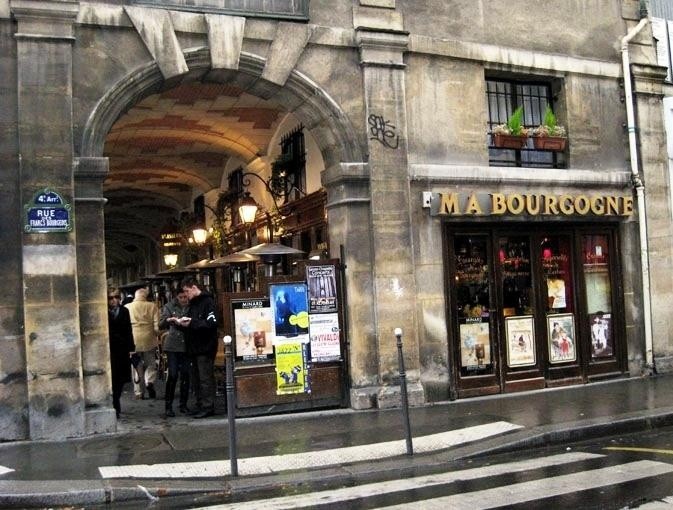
(591, 316), (608, 357)
(107, 276), (219, 419)
(551, 322), (574, 359)
(519, 335), (525, 346)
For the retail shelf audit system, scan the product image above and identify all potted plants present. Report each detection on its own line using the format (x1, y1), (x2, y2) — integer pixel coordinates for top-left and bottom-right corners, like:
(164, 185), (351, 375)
(488, 103), (568, 150)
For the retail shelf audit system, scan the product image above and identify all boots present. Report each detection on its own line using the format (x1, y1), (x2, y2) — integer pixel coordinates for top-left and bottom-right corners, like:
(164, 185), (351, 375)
(165, 401), (175, 417)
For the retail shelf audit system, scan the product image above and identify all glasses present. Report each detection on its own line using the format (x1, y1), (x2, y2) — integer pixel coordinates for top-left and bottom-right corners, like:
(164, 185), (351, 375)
(108, 295), (120, 300)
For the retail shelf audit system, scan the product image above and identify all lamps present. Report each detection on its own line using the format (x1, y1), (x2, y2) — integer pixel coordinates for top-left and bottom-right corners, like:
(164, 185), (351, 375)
(238, 171), (294, 223)
(161, 241), (180, 266)
(191, 201), (225, 246)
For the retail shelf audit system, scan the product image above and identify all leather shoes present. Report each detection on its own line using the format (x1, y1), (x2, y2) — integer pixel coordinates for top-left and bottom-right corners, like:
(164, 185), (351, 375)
(180, 404), (215, 419)
(135, 382), (156, 400)
